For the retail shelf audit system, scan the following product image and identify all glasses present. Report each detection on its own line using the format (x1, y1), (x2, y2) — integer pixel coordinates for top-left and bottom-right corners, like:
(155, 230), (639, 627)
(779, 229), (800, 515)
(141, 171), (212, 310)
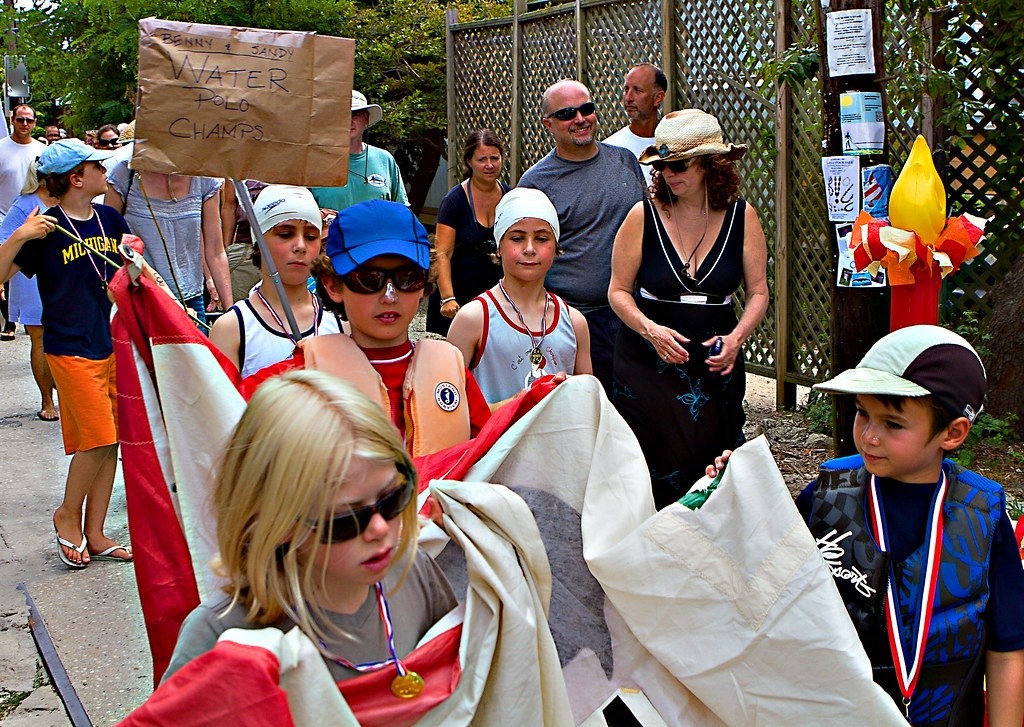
(547, 102), (594, 120)
(48, 134), (60, 137)
(652, 156), (698, 172)
(99, 138), (120, 145)
(344, 262), (427, 294)
(14, 118), (35, 124)
(298, 463), (415, 544)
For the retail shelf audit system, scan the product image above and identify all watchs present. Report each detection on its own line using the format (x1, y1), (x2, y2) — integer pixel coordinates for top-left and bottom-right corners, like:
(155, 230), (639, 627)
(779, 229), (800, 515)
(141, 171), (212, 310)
(440, 297), (455, 306)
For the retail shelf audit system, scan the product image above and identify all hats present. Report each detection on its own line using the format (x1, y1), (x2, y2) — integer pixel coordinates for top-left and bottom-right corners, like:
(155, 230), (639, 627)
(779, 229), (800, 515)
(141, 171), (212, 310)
(351, 90), (382, 130)
(636, 108), (749, 165)
(811, 324), (988, 424)
(36, 138), (115, 176)
(493, 188), (560, 244)
(325, 199), (430, 275)
(250, 182), (323, 247)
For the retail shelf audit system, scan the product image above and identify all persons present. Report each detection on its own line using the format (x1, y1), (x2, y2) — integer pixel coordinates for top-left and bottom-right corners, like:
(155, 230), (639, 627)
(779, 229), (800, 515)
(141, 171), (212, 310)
(518, 81), (651, 391)
(0, 138), (134, 567)
(209, 185), (352, 379)
(45, 121), (135, 175)
(103, 159), (268, 338)
(309, 91), (411, 221)
(445, 188), (593, 403)
(0, 156), (60, 419)
(705, 325), (1024, 727)
(159, 371), (460, 698)
(426, 129), (513, 336)
(608, 109), (769, 512)
(0, 105), (48, 339)
(602, 64), (668, 199)
(107, 199), (566, 458)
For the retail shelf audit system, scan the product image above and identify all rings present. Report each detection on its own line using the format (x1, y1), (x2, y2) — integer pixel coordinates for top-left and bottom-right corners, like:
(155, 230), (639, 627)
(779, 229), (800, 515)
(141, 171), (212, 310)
(664, 354), (670, 359)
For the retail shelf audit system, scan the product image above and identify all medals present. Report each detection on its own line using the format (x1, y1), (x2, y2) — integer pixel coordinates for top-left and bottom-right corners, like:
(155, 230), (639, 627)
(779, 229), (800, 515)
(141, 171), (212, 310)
(530, 348), (543, 363)
(391, 671), (424, 698)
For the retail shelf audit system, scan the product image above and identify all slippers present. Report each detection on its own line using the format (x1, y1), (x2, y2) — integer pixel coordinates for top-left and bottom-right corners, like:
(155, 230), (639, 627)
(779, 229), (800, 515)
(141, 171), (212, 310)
(89, 546), (133, 561)
(37, 410), (61, 421)
(1, 327), (15, 341)
(55, 524), (88, 567)
